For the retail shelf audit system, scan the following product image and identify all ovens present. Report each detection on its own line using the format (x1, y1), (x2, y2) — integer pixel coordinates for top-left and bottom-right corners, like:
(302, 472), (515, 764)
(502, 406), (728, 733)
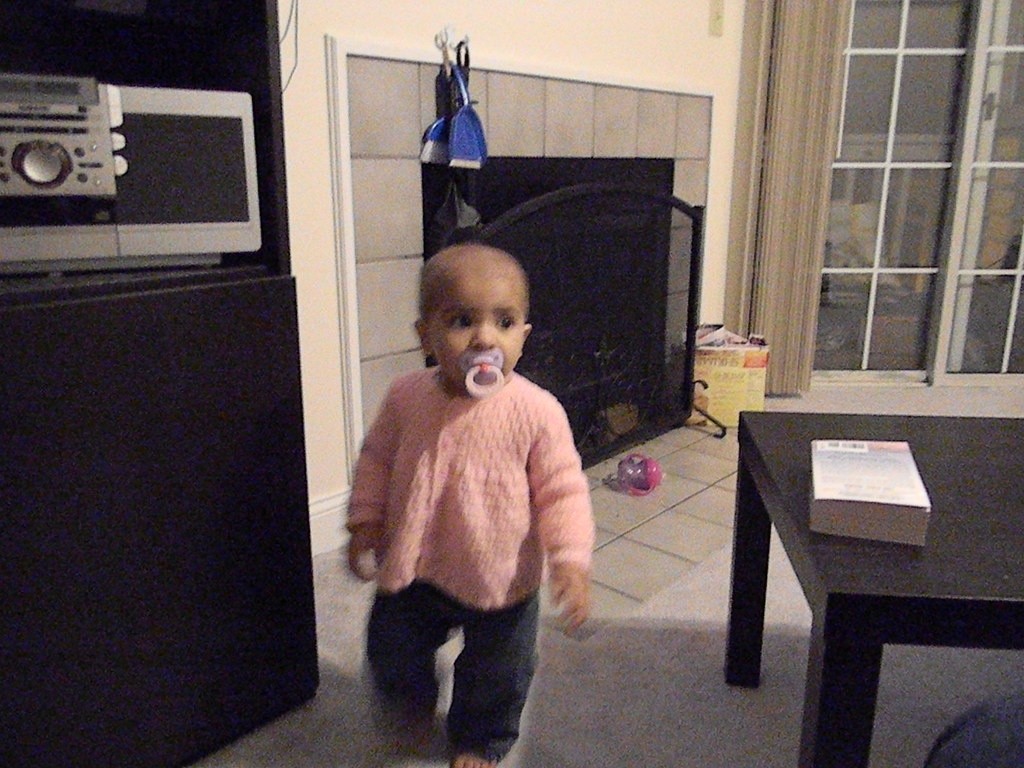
(0, 69), (264, 274)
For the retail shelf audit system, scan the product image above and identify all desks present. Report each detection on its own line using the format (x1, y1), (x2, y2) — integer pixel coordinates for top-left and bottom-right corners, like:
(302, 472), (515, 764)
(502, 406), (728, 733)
(722, 412), (1024, 768)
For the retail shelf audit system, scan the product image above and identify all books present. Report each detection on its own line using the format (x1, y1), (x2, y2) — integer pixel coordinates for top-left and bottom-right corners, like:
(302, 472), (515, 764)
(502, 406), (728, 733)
(809, 437), (933, 547)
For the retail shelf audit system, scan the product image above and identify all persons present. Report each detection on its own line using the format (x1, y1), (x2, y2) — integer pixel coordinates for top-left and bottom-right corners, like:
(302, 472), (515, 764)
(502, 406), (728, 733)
(344, 242), (594, 768)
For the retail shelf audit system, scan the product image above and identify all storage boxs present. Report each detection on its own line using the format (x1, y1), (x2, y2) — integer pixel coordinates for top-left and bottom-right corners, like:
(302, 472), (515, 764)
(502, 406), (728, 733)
(693, 344), (769, 427)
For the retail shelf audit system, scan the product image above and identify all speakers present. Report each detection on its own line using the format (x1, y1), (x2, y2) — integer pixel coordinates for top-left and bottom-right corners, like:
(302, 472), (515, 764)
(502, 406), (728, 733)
(110, 85), (261, 269)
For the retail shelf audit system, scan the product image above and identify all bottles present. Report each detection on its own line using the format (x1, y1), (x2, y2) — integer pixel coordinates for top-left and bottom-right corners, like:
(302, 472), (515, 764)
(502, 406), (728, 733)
(618, 453), (666, 496)
